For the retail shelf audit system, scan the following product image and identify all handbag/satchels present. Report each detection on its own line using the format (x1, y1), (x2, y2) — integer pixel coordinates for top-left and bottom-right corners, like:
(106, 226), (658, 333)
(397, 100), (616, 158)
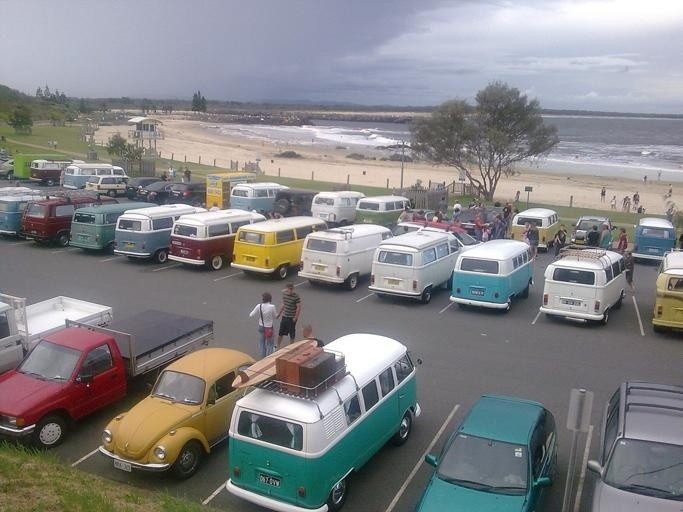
(263, 327), (273, 337)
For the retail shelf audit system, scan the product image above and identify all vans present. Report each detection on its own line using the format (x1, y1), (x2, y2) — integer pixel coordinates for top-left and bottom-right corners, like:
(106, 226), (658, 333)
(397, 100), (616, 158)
(222, 328), (423, 511)
(646, 246), (682, 337)
(536, 247), (628, 329)
(628, 215), (676, 266)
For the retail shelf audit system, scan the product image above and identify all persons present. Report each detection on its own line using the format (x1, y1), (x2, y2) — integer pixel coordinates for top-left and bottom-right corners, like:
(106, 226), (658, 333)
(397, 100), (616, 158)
(599, 223), (612, 249)
(616, 226), (629, 252)
(555, 224), (567, 257)
(302, 324), (325, 348)
(407, 190), (541, 259)
(250, 207), (284, 220)
(624, 250), (638, 293)
(161, 163), (177, 181)
(275, 282), (303, 351)
(250, 292), (280, 359)
(587, 225), (600, 247)
(183, 166), (191, 183)
(208, 201), (220, 212)
(601, 168), (673, 225)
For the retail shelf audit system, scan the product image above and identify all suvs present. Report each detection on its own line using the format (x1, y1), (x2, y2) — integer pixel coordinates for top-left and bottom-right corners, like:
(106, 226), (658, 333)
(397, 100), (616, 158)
(584, 378), (682, 512)
(568, 214), (618, 252)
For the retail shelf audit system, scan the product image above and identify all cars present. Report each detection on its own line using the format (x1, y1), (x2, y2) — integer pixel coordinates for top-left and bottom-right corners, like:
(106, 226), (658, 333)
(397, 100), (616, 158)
(410, 389), (567, 512)
(78, 344), (265, 481)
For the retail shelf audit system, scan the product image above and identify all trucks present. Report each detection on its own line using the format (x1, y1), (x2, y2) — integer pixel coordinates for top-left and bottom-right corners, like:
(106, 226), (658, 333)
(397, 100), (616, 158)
(0, 292), (116, 376)
(0, 309), (220, 451)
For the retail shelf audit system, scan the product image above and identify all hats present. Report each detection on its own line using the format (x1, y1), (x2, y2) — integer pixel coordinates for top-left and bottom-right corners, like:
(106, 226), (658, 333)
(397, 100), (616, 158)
(493, 214), (501, 219)
(286, 283), (294, 289)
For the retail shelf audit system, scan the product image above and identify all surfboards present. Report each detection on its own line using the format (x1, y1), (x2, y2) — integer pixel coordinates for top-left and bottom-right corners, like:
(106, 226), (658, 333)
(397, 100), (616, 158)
(231, 339), (318, 389)
